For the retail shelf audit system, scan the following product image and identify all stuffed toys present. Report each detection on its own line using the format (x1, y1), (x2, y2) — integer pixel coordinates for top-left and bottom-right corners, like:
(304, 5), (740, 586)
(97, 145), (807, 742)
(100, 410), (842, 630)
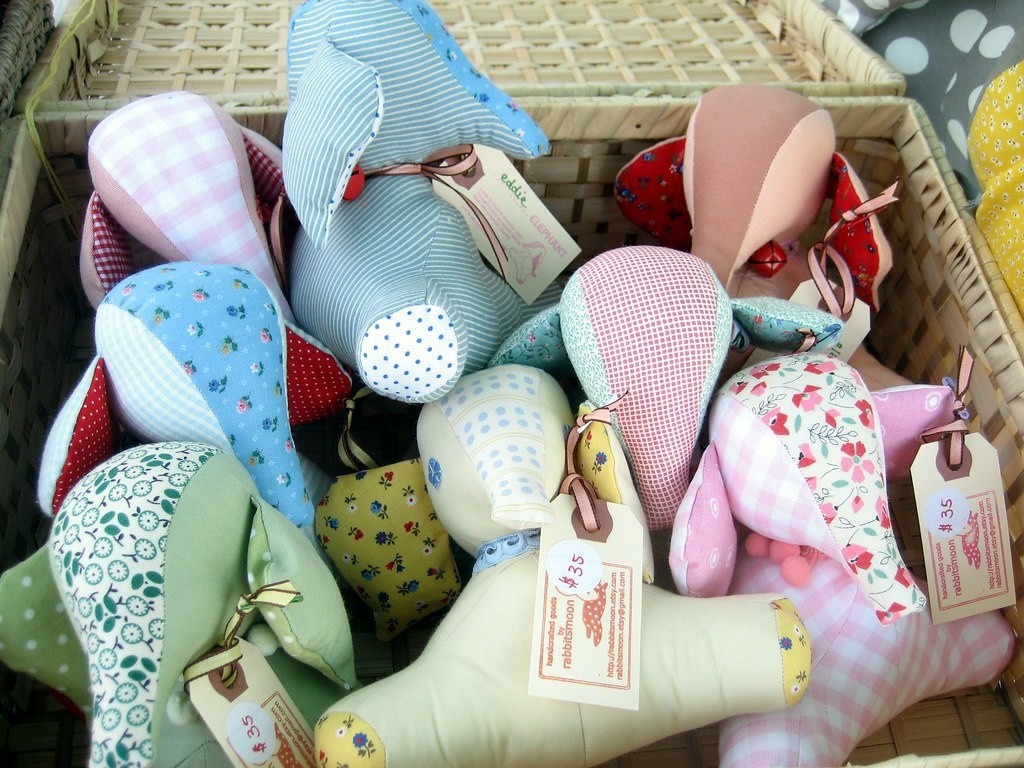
(0, 0), (1011, 768)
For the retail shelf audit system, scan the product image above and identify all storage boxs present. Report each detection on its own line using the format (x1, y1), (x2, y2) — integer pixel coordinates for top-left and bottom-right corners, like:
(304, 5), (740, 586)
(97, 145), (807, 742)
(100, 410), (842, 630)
(0, 0), (1024, 768)
